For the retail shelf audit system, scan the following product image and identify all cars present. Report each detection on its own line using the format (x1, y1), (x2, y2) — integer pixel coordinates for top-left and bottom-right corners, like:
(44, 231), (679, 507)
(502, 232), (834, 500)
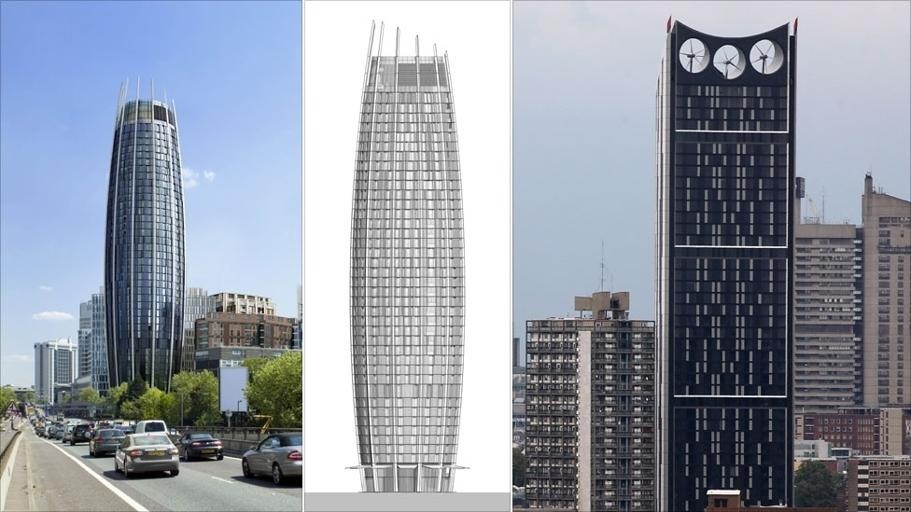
(241, 432), (302, 486)
(30, 413), (224, 480)
